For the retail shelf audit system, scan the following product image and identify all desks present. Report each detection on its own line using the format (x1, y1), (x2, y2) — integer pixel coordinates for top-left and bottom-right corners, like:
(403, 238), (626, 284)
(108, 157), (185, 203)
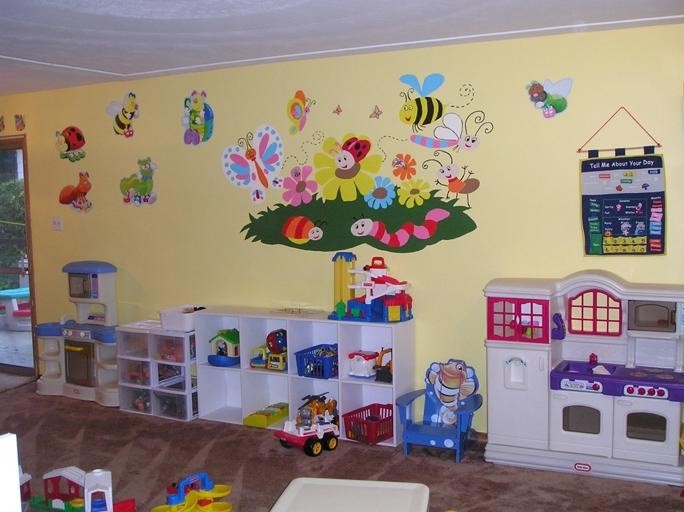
(267, 474), (431, 512)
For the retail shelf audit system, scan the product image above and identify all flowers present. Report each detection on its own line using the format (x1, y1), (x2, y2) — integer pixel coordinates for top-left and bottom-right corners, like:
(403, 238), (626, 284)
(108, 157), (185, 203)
(280, 129), (430, 213)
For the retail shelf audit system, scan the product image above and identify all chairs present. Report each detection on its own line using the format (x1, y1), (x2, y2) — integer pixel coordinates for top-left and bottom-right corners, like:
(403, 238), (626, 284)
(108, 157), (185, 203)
(394, 358), (483, 464)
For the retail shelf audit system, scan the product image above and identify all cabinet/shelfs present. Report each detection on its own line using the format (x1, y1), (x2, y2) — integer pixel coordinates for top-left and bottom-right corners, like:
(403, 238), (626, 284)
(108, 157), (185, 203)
(116, 320), (198, 423)
(195, 306), (413, 453)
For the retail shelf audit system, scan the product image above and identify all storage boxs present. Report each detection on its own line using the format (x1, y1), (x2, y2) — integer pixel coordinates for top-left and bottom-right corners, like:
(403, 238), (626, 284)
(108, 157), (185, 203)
(156, 303), (205, 332)
(346, 350), (379, 379)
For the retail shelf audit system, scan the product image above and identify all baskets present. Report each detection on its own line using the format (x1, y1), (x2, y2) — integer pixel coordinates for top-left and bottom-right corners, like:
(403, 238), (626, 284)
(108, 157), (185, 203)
(294, 343), (338, 379)
(341, 403), (393, 446)
